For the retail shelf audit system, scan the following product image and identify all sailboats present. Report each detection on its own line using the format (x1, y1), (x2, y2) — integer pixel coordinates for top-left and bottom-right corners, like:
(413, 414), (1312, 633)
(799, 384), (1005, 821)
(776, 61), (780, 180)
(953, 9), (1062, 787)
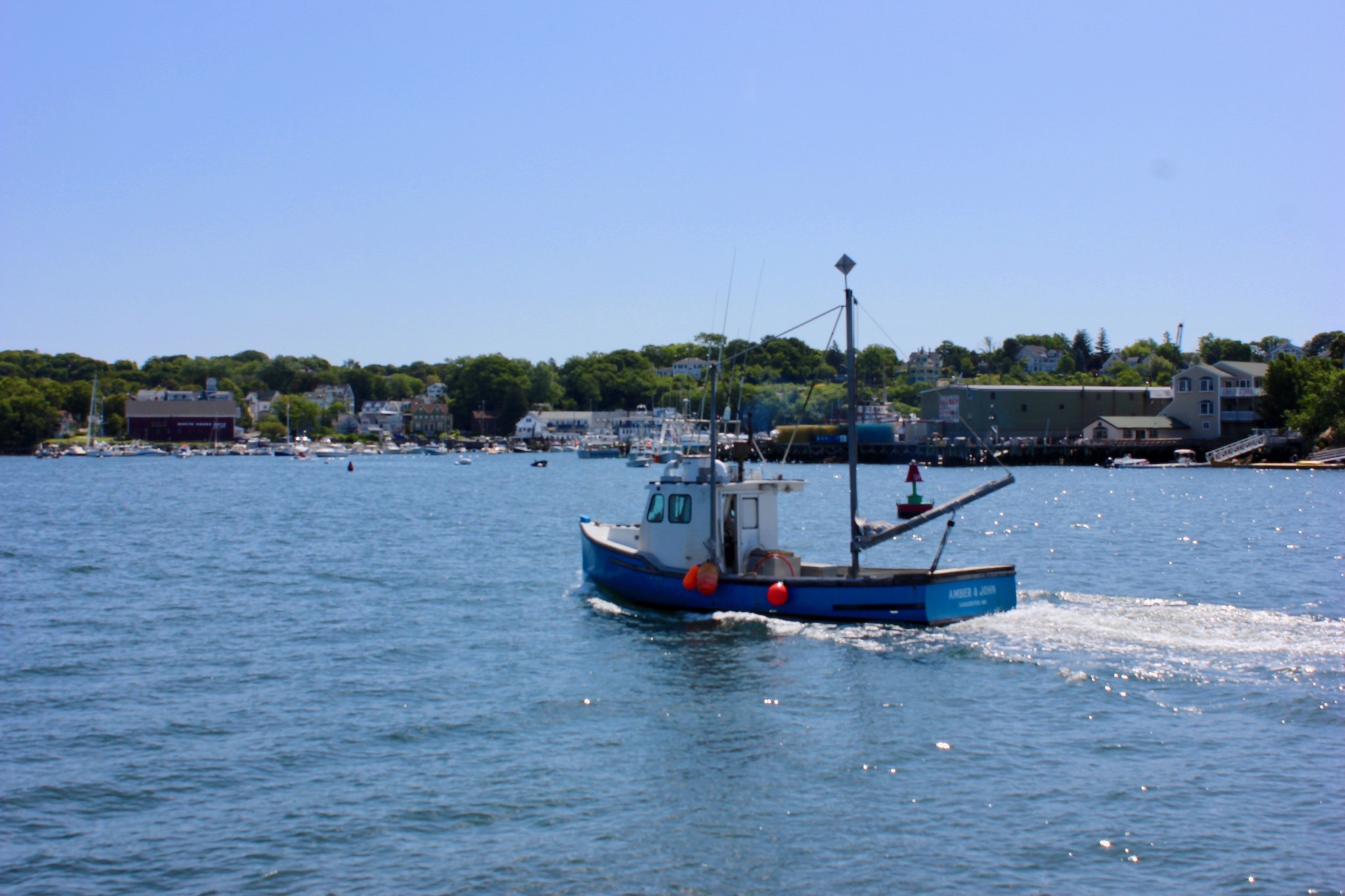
(249, 390), (275, 456)
(275, 402), (296, 457)
(85, 372), (113, 457)
(206, 408), (228, 456)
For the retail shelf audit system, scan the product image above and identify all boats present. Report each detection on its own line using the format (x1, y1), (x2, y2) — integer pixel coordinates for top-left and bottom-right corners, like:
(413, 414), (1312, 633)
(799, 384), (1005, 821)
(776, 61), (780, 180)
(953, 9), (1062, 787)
(482, 442), (532, 454)
(125, 440), (169, 457)
(311, 437), (350, 456)
(1111, 453), (1149, 467)
(350, 438), (400, 455)
(64, 444), (85, 456)
(580, 252), (1015, 629)
(230, 444), (247, 455)
(401, 443), (422, 454)
(550, 445), (577, 454)
(295, 443), (310, 455)
(101, 444), (125, 457)
(425, 438), (447, 455)
(178, 445), (193, 458)
(581, 418), (682, 467)
(461, 458), (472, 464)
